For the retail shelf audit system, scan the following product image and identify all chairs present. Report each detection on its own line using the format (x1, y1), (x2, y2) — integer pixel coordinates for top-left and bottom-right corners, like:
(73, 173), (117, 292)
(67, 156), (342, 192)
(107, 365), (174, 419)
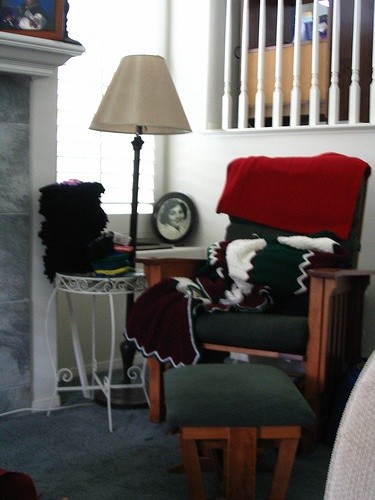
(136, 152), (375, 423)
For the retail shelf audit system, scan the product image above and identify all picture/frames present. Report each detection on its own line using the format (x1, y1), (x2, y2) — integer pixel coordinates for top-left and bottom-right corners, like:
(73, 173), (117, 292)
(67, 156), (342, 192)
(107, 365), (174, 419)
(152, 192), (198, 244)
(0, 0), (65, 42)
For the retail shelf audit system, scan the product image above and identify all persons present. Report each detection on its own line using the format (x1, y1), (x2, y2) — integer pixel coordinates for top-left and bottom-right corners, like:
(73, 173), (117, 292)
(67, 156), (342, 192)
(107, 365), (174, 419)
(160, 200), (187, 239)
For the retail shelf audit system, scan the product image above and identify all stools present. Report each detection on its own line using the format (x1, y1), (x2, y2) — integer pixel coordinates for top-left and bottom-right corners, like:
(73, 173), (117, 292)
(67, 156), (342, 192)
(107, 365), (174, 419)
(164, 364), (318, 500)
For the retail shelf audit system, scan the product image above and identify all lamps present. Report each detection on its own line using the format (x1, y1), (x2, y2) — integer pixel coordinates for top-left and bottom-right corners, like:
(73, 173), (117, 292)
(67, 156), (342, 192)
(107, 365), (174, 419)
(89, 56), (192, 409)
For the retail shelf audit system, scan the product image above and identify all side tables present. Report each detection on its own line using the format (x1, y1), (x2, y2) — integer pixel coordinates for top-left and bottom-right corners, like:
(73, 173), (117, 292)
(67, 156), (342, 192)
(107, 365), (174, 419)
(135, 246), (206, 259)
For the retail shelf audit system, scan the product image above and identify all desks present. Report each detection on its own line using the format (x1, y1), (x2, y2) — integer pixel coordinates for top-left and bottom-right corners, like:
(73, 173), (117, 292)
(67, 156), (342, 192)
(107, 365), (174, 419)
(247, 39), (328, 119)
